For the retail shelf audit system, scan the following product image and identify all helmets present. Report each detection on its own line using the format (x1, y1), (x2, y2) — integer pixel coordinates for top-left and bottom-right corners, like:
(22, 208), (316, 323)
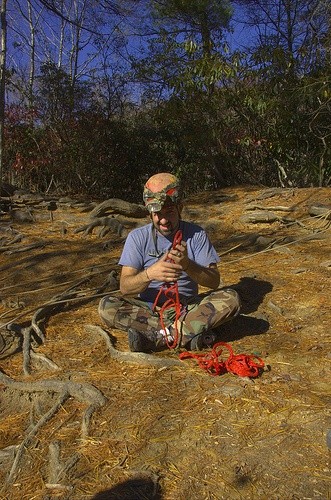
(142, 172), (185, 211)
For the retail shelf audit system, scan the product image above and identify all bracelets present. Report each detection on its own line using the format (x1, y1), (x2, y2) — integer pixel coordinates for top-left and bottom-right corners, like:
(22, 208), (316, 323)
(145, 267), (152, 283)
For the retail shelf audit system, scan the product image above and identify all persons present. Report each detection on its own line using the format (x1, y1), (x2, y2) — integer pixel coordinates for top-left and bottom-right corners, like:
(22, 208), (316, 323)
(98, 173), (243, 351)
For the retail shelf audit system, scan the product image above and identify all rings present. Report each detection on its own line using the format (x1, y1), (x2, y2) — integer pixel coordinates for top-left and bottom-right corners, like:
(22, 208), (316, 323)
(177, 252), (181, 257)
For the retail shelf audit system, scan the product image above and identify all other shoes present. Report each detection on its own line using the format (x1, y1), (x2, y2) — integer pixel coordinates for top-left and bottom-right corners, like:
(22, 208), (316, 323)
(127, 327), (165, 353)
(185, 332), (204, 352)
